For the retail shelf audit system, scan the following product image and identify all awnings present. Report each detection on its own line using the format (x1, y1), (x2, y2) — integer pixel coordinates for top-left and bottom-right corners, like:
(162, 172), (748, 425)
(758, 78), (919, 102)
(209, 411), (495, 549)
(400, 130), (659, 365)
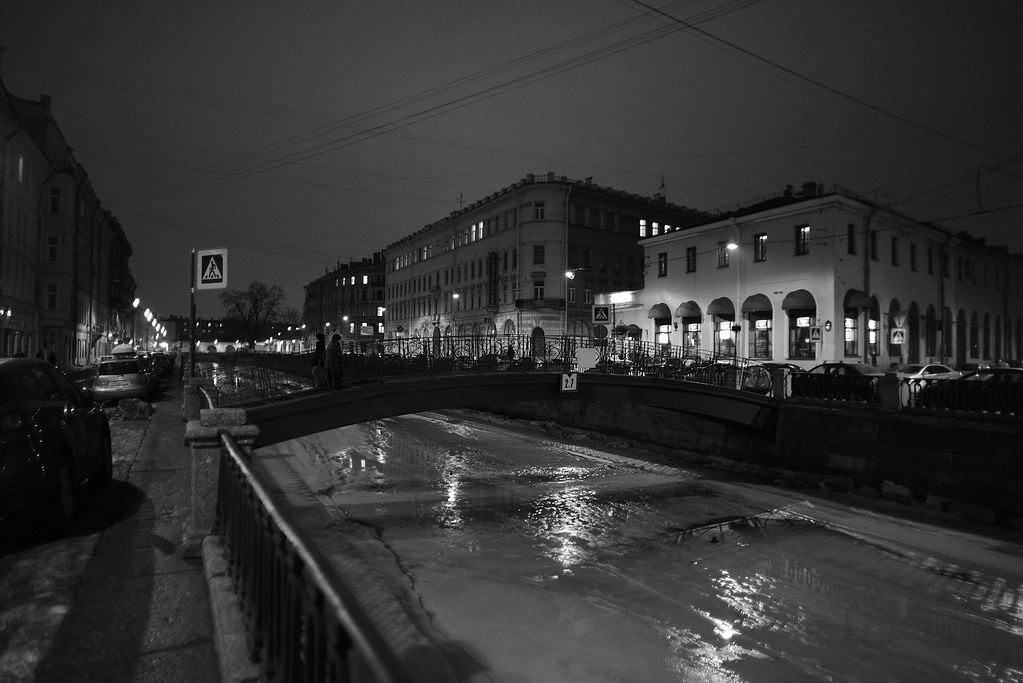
(706, 297), (734, 315)
(611, 324), (642, 334)
(111, 343), (137, 357)
(742, 294), (773, 313)
(844, 289), (875, 313)
(674, 302), (700, 318)
(782, 289), (816, 310)
(648, 303), (671, 319)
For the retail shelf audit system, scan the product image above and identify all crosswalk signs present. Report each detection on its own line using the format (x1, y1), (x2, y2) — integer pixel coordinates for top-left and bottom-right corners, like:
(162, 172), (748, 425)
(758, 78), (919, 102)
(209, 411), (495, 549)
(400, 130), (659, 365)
(195, 248), (228, 290)
(592, 304), (611, 324)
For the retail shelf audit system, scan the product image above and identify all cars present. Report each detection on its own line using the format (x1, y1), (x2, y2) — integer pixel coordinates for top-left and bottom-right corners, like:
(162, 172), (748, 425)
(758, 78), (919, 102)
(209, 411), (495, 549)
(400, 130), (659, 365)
(740, 363), (808, 389)
(888, 362), (962, 405)
(476, 353), (511, 372)
(538, 357), (578, 372)
(90, 358), (158, 403)
(342, 350), (474, 372)
(804, 360), (887, 401)
(507, 355), (549, 371)
(135, 349), (177, 373)
(953, 366), (1023, 393)
(578, 354), (745, 389)
(0, 356), (114, 536)
(949, 359), (1023, 386)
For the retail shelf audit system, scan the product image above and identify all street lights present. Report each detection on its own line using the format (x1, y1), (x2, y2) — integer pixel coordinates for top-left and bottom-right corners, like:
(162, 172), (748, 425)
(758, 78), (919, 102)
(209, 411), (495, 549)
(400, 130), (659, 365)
(732, 324), (741, 368)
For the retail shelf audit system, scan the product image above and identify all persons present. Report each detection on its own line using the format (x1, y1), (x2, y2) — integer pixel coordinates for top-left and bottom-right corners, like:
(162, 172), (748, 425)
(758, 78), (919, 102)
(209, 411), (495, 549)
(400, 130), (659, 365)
(169, 350), (176, 364)
(310, 333), (345, 390)
(36, 350), (58, 366)
(1, 348), (26, 358)
